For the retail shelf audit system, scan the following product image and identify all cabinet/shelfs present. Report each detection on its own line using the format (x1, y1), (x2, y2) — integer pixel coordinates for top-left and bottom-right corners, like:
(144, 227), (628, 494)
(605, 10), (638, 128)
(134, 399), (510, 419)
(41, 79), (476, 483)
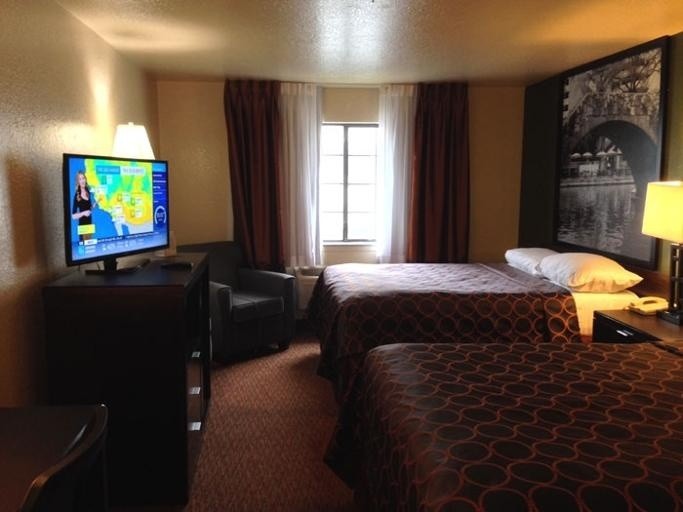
(41, 251), (211, 506)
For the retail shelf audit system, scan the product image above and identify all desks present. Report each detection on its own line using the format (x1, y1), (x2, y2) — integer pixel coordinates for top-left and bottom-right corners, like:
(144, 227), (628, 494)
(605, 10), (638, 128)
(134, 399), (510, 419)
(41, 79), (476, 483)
(2, 403), (99, 512)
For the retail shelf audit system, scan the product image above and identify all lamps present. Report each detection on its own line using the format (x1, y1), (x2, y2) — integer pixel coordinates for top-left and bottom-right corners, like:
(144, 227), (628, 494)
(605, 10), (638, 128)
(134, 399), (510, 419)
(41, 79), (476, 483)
(642, 179), (683, 327)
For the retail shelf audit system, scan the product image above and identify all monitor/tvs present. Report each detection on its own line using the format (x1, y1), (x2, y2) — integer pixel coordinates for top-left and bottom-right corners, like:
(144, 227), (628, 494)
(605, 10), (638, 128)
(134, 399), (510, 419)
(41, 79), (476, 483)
(62, 152), (170, 275)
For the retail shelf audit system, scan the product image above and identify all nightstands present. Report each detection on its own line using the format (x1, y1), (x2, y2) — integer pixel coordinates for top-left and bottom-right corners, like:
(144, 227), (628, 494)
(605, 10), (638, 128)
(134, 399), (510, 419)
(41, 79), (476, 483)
(592, 310), (683, 344)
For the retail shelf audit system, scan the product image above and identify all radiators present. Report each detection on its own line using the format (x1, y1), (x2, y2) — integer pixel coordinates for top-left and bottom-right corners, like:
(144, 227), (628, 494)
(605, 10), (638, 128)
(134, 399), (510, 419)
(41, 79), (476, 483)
(292, 265), (325, 310)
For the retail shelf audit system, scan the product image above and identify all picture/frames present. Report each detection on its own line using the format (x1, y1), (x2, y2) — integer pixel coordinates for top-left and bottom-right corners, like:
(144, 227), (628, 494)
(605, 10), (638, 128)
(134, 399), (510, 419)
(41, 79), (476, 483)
(551, 34), (674, 272)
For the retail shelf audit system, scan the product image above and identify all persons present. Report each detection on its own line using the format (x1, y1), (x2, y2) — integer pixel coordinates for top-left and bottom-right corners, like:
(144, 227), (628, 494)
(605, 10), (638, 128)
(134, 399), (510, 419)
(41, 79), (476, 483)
(72, 171), (96, 245)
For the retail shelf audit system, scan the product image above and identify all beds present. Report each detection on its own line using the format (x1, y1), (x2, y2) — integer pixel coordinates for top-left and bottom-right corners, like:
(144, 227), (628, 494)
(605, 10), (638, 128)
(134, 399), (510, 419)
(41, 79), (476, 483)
(319, 340), (683, 512)
(302, 262), (643, 408)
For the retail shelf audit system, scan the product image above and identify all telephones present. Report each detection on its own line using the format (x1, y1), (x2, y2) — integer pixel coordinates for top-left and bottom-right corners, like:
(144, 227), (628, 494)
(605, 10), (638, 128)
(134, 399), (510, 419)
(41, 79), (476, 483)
(628, 296), (670, 316)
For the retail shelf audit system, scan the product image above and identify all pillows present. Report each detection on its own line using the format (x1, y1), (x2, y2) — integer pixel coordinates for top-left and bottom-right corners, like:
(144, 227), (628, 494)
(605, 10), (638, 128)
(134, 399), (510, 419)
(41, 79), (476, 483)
(505, 246), (560, 278)
(535, 252), (644, 295)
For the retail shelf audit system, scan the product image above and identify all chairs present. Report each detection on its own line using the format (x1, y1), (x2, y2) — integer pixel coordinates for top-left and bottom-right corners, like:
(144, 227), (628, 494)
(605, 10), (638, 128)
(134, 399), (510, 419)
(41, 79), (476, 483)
(18, 403), (110, 512)
(175, 240), (297, 366)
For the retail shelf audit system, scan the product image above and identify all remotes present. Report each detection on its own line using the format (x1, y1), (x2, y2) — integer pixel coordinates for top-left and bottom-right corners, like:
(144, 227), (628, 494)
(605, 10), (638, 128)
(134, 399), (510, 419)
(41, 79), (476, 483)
(174, 259), (194, 269)
